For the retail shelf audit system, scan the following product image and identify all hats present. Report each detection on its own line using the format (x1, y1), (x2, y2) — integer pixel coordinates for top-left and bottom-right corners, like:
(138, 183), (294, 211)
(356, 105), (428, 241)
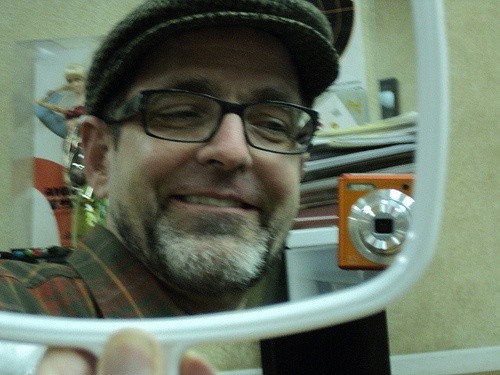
(85, 0), (356, 114)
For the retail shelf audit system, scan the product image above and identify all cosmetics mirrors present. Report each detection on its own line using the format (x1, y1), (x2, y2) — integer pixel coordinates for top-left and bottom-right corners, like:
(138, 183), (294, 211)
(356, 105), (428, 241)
(0, 0), (448, 374)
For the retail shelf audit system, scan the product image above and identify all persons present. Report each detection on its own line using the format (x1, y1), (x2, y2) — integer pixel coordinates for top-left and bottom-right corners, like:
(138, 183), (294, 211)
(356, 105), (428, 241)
(0, 0), (354, 319)
(36, 328), (215, 374)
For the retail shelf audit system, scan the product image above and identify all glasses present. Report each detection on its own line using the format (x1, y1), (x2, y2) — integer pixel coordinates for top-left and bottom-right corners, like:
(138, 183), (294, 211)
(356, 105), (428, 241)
(103, 88), (321, 155)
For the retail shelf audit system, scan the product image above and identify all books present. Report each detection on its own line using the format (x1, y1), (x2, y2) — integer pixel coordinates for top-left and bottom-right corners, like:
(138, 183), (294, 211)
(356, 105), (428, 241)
(301, 111), (420, 210)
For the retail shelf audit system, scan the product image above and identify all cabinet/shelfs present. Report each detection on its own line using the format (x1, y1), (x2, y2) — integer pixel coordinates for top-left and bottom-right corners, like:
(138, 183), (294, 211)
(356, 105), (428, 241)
(285, 245), (384, 301)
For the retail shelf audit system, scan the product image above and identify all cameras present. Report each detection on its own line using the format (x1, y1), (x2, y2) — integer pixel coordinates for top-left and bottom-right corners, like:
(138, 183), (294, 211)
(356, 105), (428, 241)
(338, 173), (414, 270)
